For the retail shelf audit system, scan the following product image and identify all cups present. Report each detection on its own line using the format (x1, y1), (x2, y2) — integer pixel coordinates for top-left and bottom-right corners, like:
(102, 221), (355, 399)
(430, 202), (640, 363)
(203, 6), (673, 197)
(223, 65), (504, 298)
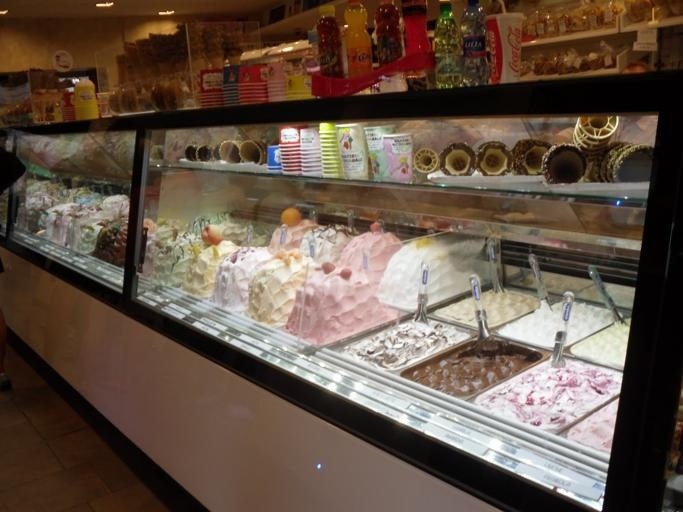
(60, 78), (99, 121)
(482, 12), (527, 87)
(196, 61), (314, 109)
(264, 121), (414, 185)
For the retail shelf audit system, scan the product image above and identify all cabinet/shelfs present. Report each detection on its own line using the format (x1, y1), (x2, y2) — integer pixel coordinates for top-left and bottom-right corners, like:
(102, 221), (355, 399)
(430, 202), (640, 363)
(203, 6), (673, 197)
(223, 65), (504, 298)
(520, 16), (683, 83)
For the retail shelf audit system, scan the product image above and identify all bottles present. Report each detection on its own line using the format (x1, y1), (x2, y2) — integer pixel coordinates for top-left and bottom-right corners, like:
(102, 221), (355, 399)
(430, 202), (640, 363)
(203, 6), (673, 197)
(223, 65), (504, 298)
(316, 0), (489, 91)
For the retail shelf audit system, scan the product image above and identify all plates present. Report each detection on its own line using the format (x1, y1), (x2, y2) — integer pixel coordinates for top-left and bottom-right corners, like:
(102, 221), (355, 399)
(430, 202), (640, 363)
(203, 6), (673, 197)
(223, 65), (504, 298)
(547, 181), (651, 192)
(425, 170), (545, 185)
(176, 159), (265, 171)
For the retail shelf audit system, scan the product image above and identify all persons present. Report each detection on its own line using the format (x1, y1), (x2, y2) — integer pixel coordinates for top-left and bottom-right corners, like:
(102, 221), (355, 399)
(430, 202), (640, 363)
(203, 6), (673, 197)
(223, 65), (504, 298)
(0, 129), (28, 391)
